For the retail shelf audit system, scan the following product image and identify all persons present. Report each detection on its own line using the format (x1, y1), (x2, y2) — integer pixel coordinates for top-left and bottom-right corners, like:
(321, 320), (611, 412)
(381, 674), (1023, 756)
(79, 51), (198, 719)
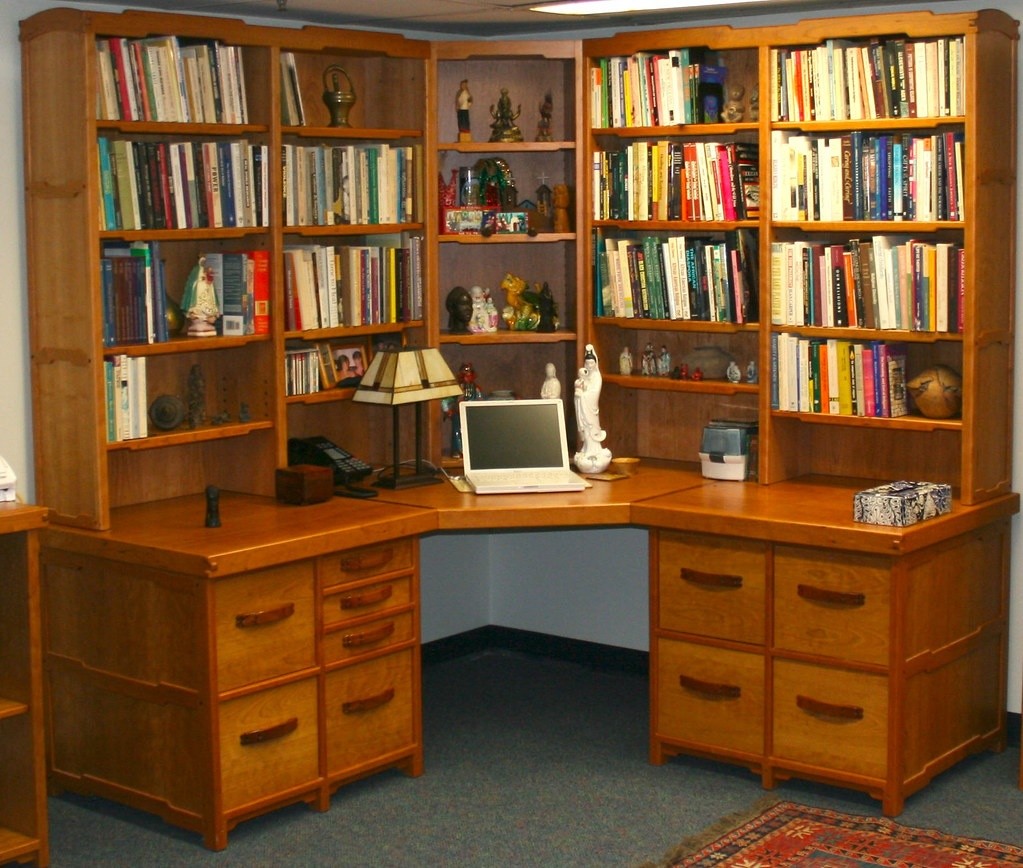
(466, 285), (499, 334)
(573, 344), (606, 455)
(454, 79), (473, 134)
(537, 361), (562, 400)
(445, 287), (474, 335)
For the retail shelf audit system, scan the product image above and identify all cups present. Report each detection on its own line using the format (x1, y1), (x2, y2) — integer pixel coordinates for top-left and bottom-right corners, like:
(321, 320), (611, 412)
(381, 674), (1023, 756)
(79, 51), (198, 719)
(612, 457), (641, 476)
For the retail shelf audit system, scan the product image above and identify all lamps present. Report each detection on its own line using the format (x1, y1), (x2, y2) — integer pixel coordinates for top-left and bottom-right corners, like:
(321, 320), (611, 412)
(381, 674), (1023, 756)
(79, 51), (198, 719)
(350, 344), (464, 489)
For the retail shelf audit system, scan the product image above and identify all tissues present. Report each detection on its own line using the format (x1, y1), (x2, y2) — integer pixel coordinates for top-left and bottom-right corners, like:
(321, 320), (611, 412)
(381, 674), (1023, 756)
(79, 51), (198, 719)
(852, 476), (956, 529)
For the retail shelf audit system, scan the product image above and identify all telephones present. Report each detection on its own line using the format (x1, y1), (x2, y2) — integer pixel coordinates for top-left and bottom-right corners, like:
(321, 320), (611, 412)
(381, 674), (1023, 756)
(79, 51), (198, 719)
(286, 434), (371, 481)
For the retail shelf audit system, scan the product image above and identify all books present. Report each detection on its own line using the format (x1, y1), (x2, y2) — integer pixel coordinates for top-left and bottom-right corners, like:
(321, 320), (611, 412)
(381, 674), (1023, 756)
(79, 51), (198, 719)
(91, 31), (427, 447)
(587, 37), (969, 426)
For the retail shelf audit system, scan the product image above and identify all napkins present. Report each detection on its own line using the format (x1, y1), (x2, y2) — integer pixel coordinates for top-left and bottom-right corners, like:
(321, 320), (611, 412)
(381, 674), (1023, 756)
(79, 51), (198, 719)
(852, 479), (953, 527)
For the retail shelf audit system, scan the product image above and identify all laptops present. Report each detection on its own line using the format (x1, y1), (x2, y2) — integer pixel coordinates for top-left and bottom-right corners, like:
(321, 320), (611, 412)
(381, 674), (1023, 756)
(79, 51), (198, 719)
(460, 400), (585, 495)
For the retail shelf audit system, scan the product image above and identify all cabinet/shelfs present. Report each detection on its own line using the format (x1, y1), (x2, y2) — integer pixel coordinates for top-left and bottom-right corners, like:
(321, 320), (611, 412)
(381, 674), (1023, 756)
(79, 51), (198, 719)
(631, 470), (1019, 817)
(24, 5), (1018, 529)
(0, 501), (50, 866)
(34, 490), (438, 853)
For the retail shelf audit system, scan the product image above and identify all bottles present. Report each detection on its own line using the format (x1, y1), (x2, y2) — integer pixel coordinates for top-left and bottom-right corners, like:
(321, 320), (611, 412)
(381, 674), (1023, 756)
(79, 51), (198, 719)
(485, 390), (515, 401)
(727, 362), (740, 384)
(748, 361), (757, 384)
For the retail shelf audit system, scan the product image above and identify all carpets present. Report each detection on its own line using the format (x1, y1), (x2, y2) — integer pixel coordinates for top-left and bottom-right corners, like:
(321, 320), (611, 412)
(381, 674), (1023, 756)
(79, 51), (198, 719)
(641, 790), (1023, 868)
